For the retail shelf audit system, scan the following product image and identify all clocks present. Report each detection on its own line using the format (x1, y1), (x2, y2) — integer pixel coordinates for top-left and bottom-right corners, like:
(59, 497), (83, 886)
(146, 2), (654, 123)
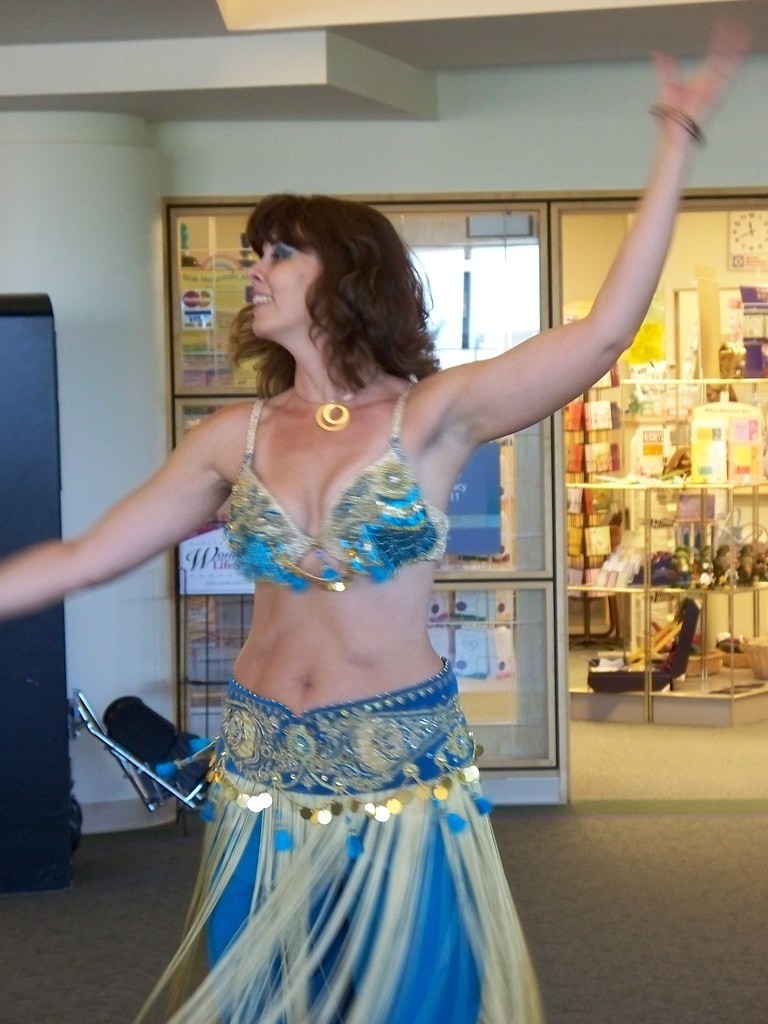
(727, 211), (768, 273)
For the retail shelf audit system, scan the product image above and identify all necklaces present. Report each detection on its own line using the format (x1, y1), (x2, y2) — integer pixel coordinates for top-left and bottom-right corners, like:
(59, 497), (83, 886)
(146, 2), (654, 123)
(292, 385), (356, 432)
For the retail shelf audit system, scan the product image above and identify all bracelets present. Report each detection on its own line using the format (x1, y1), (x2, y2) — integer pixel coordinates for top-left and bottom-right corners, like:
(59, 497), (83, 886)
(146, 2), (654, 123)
(648, 102), (706, 150)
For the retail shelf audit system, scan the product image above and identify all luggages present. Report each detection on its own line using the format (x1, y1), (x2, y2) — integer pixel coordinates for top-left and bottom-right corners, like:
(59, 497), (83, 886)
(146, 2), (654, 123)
(585, 597), (701, 692)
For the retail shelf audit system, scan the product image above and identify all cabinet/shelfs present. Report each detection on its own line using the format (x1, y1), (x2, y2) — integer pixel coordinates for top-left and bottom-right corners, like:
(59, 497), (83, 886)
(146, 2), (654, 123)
(566, 481), (768, 728)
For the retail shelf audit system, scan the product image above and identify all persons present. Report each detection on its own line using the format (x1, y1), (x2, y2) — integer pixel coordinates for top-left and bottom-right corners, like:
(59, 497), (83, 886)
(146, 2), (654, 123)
(0, 6), (754, 1024)
